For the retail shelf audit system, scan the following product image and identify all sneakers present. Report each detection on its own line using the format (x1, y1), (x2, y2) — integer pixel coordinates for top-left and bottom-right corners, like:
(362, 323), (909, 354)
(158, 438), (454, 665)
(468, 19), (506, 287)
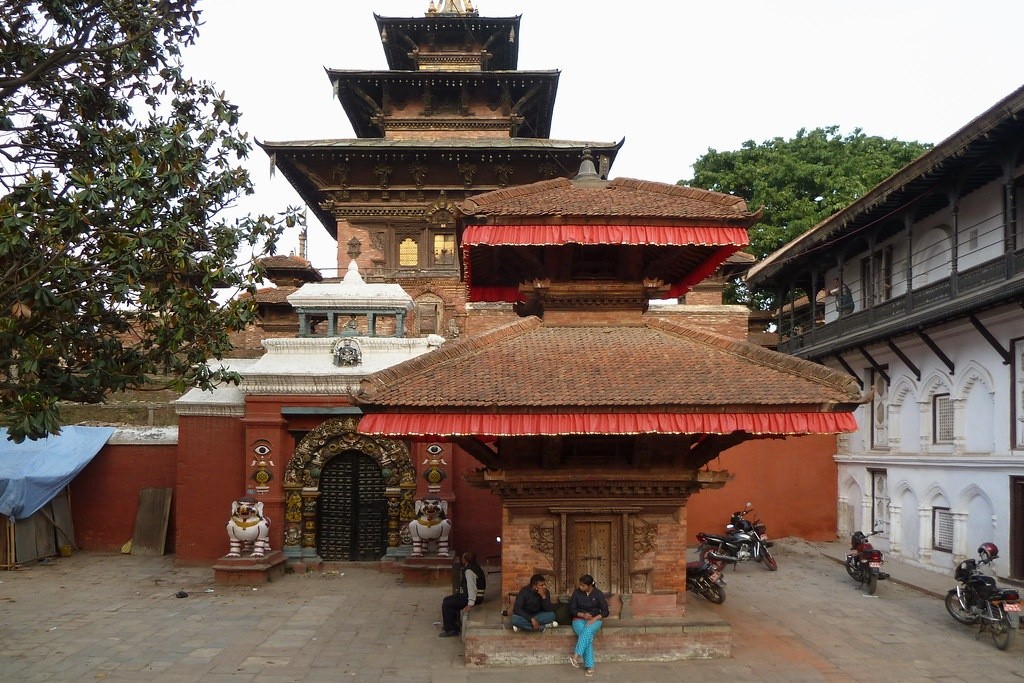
(513, 625), (521, 632)
(545, 621), (558, 628)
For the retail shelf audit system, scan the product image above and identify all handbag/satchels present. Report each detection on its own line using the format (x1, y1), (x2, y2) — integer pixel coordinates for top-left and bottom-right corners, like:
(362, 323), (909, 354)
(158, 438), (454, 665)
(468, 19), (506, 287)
(553, 604), (572, 625)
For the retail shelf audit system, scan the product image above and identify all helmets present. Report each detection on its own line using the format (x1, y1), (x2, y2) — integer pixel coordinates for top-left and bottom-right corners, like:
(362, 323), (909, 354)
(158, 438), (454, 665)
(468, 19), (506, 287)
(978, 542), (998, 560)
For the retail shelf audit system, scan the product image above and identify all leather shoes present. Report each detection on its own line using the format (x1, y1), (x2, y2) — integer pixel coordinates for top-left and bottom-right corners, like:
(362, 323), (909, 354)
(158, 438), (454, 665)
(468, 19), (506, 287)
(439, 629), (459, 637)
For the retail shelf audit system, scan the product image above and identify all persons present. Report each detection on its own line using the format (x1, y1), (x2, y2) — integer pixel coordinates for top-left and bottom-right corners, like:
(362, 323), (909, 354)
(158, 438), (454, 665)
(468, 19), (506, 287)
(511, 575), (558, 632)
(570, 575), (609, 676)
(439, 552), (485, 637)
(824, 278), (854, 317)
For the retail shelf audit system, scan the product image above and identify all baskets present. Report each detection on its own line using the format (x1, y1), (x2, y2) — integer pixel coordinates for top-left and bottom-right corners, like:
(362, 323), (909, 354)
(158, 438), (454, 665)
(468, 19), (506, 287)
(756, 524), (766, 534)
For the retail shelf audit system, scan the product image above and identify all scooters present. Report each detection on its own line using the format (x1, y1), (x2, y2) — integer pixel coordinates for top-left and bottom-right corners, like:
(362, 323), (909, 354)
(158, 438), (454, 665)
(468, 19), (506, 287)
(686, 561), (727, 605)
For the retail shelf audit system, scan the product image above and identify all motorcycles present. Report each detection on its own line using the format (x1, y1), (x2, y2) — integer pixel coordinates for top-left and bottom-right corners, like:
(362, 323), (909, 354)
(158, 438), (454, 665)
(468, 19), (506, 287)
(725, 502), (768, 562)
(945, 553), (1021, 651)
(845, 520), (891, 595)
(695, 514), (778, 572)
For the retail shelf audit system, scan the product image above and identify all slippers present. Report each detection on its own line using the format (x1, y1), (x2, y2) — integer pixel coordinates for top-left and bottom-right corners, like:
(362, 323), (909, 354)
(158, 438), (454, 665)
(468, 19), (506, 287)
(570, 657), (579, 667)
(585, 669), (594, 676)
